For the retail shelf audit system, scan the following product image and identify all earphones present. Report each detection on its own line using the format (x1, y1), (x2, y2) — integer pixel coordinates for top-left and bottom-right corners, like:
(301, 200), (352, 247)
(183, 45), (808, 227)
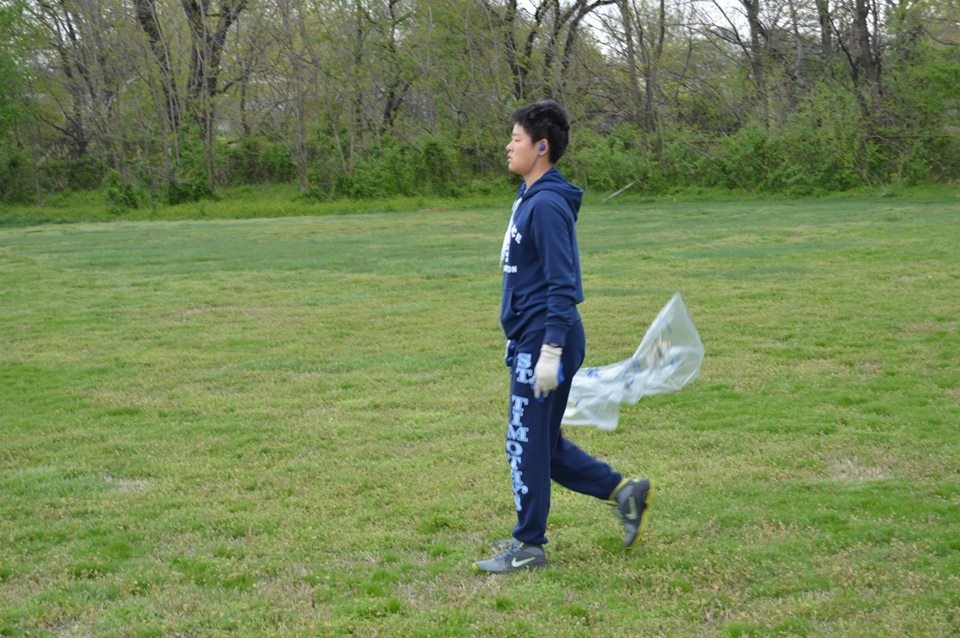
(539, 144), (546, 151)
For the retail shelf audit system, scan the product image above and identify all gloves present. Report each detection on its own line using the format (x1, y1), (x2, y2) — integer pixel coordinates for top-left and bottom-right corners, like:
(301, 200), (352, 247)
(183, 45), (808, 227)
(529, 344), (564, 399)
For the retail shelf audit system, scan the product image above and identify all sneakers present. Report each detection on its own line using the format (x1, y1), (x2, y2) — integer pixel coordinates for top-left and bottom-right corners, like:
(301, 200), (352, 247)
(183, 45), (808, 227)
(617, 477), (650, 548)
(475, 545), (546, 573)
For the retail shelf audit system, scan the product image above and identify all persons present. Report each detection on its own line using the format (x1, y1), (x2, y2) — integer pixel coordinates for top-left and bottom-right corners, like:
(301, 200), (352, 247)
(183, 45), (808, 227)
(472, 101), (650, 574)
